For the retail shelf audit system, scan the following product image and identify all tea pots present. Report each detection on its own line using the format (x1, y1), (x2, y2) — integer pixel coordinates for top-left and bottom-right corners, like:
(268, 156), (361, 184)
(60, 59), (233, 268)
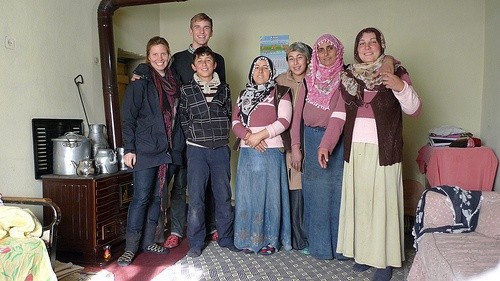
(71, 156), (101, 177)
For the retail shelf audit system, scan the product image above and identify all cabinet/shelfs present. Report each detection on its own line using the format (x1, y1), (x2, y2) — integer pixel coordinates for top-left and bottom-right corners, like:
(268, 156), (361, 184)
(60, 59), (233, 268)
(41, 171), (134, 260)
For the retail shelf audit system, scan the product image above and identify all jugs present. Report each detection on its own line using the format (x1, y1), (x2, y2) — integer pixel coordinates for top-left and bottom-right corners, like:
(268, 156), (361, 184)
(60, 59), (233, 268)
(95, 148), (118, 174)
(116, 145), (128, 170)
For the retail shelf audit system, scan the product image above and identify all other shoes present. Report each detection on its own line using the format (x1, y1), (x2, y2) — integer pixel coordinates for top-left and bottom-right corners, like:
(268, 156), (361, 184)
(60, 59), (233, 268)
(260, 245), (274, 255)
(117, 251), (136, 266)
(208, 232), (218, 240)
(163, 234), (183, 248)
(145, 243), (169, 254)
(353, 263), (370, 271)
(372, 267), (391, 281)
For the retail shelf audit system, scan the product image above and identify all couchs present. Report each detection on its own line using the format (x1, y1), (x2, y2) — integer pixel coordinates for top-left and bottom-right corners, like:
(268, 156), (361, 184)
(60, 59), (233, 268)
(407, 192), (500, 281)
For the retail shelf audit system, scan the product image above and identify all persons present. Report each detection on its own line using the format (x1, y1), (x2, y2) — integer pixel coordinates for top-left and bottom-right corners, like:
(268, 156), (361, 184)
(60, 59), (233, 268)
(132, 12), (226, 249)
(179, 45), (242, 257)
(232, 55), (291, 253)
(290, 33), (395, 259)
(273, 42), (313, 254)
(317, 29), (422, 281)
(117, 36), (185, 266)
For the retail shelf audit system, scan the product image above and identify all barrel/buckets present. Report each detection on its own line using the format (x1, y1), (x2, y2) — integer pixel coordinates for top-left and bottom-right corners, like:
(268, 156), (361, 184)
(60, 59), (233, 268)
(51, 132), (92, 175)
(88, 124), (110, 158)
